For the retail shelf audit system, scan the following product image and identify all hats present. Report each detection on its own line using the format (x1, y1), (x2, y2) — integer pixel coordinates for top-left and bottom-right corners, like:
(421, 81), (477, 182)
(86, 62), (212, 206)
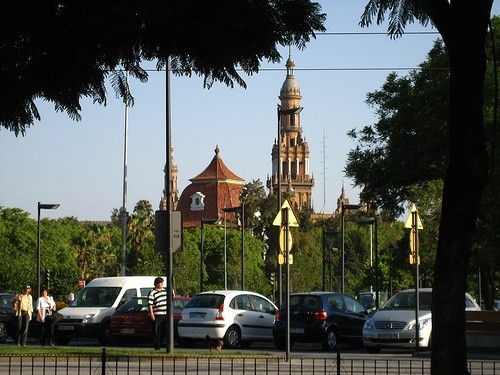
(23, 284), (30, 288)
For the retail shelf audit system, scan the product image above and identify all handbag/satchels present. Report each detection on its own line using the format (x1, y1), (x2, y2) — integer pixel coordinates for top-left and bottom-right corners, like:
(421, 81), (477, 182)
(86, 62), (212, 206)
(11, 293), (21, 312)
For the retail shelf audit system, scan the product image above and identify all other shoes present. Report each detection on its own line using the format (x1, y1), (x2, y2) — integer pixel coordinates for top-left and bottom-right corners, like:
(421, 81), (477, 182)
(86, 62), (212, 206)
(158, 348), (166, 353)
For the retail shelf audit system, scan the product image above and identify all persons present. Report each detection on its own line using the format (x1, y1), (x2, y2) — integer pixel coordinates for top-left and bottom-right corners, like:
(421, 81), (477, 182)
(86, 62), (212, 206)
(148, 278), (171, 351)
(15, 284), (75, 348)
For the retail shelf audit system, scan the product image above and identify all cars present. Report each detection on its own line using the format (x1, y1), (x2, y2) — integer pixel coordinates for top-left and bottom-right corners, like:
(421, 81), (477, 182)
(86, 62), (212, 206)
(177, 289), (278, 347)
(0, 293), (44, 343)
(272, 291), (372, 351)
(363, 288), (483, 353)
(112, 297), (192, 346)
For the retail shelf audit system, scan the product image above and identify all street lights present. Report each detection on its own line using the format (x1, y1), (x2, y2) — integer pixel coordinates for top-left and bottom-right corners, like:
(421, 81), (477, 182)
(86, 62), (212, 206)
(200, 216), (218, 290)
(357, 216), (379, 308)
(340, 201), (362, 291)
(221, 201), (244, 290)
(322, 228), (338, 289)
(36, 201), (61, 298)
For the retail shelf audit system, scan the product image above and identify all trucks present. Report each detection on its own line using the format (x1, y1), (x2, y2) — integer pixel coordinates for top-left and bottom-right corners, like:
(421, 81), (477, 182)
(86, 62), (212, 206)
(53, 277), (167, 346)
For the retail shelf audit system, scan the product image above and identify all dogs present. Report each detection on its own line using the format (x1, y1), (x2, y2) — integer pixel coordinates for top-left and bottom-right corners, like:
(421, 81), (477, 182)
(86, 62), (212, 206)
(204, 333), (225, 355)
